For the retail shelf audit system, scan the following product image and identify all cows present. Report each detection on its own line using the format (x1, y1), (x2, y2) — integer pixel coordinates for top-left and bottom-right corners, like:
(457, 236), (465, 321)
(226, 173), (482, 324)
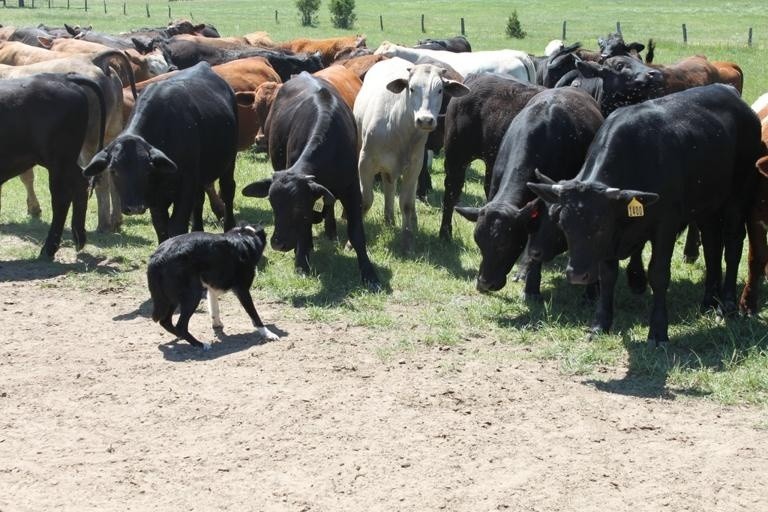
(146, 224), (275, 351)
(441, 67), (538, 232)
(528, 85), (766, 343)
(457, 91), (602, 292)
(0, 75), (106, 263)
(83, 62), (242, 236)
(0, 18), (749, 222)
(242, 78), (374, 279)
(353, 57), (462, 230)
